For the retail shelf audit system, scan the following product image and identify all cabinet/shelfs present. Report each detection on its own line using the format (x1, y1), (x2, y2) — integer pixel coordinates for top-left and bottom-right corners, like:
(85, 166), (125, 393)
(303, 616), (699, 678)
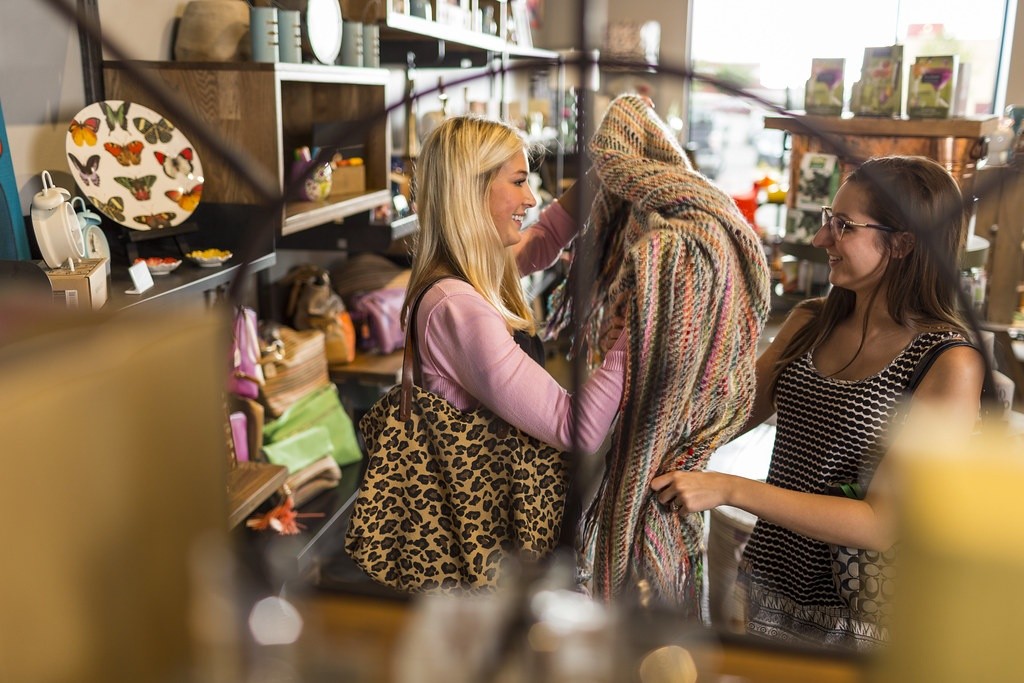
(769, 112), (1002, 311)
(80, 1), (560, 587)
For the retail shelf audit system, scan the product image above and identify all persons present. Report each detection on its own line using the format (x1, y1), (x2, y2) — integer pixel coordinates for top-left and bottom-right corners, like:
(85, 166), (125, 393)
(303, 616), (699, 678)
(649, 155), (988, 654)
(400, 116), (632, 594)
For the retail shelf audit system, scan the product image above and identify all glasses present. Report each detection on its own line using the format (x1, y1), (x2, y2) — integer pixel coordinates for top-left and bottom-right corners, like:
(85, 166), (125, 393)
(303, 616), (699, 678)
(821, 205), (901, 241)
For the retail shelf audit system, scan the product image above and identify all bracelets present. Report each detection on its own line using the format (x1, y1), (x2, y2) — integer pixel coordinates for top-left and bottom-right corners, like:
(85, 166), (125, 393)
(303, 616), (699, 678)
(584, 168), (598, 194)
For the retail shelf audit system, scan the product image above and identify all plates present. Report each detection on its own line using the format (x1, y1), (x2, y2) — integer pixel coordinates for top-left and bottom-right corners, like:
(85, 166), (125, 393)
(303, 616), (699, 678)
(65, 100), (204, 231)
(133, 260), (182, 275)
(187, 254), (233, 267)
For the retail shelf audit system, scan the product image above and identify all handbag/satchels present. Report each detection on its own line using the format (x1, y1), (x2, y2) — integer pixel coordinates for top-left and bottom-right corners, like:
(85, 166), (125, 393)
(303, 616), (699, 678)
(282, 265), (355, 365)
(263, 381), (364, 472)
(343, 276), (568, 600)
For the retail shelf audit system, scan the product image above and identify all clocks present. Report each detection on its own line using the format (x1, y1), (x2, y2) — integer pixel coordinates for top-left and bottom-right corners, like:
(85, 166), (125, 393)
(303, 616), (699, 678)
(30, 170), (86, 272)
(70, 196), (111, 276)
